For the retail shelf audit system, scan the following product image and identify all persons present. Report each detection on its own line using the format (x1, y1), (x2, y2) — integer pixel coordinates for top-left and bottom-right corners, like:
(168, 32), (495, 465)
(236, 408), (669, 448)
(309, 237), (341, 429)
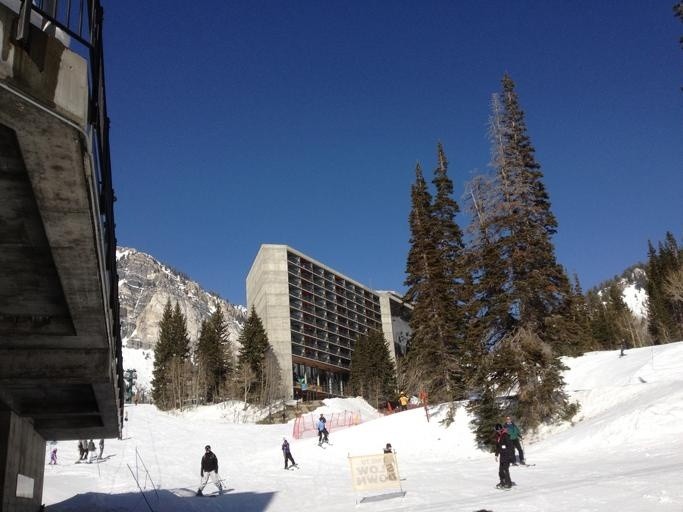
(503, 415), (526, 465)
(281, 438), (296, 470)
(318, 418), (328, 442)
(383, 443), (397, 482)
(77, 437), (105, 461)
(318, 414), (329, 436)
(48, 448), (58, 464)
(195, 446), (225, 497)
(398, 391), (409, 411)
(494, 423), (515, 489)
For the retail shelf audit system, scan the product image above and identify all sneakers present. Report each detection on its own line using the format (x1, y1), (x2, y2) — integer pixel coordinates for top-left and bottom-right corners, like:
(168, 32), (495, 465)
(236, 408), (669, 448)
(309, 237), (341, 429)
(513, 461), (519, 466)
(520, 458), (525, 465)
(496, 480), (511, 489)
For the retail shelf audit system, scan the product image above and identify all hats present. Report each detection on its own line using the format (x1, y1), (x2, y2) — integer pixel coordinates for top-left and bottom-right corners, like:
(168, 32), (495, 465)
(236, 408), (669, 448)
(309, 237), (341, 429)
(495, 424), (502, 430)
